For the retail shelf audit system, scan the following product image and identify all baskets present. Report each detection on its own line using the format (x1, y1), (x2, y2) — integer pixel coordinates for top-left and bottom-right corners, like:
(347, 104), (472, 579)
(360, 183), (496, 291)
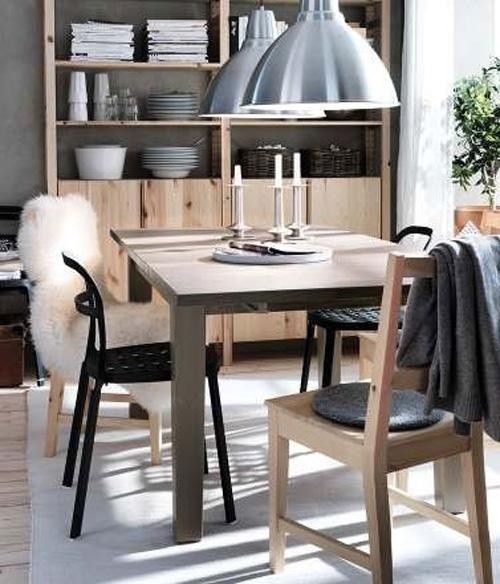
(300, 147), (363, 177)
(237, 149), (293, 178)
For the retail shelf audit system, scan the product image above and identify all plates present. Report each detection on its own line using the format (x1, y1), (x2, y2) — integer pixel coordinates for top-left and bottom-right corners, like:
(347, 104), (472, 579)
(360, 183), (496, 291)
(237, 147), (291, 177)
(146, 92), (201, 120)
(302, 148), (363, 177)
(141, 145), (200, 178)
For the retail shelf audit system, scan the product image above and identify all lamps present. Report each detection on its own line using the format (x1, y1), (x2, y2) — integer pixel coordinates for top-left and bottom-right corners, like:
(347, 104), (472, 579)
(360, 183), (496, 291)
(241, 0), (402, 111)
(197, 0), (325, 118)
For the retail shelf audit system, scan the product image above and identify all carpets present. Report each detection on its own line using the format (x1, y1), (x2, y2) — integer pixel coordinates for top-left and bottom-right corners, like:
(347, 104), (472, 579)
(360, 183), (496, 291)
(27, 376), (500, 584)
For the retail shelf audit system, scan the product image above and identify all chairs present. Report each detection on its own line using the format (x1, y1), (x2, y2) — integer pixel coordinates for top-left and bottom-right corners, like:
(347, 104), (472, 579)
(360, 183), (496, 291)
(299, 226), (433, 391)
(264, 239), (500, 581)
(15, 192), (175, 464)
(58, 252), (239, 538)
(1, 201), (49, 385)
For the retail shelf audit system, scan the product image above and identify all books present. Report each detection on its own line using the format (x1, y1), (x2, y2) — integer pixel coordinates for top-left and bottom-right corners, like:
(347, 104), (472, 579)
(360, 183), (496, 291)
(230, 14), (290, 56)
(347, 18), (377, 52)
(70, 18), (135, 63)
(143, 17), (212, 64)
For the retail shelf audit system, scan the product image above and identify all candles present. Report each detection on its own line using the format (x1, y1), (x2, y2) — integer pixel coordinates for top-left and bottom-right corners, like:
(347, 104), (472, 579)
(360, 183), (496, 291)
(234, 163), (241, 186)
(293, 152), (301, 185)
(275, 154), (283, 186)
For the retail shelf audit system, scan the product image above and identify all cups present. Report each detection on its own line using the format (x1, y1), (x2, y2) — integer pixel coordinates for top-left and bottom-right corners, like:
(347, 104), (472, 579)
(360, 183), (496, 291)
(67, 71), (88, 103)
(93, 73), (110, 104)
(67, 103), (88, 121)
(93, 105), (110, 121)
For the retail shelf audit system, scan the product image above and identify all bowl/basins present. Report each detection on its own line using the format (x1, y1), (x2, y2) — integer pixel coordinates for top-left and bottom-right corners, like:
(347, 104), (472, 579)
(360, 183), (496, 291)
(73, 144), (127, 180)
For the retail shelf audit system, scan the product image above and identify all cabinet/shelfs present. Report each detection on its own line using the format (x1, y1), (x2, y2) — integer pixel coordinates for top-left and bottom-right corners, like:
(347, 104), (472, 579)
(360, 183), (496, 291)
(43, 0), (226, 180)
(48, 180), (229, 366)
(226, 178), (396, 367)
(224, 0), (387, 178)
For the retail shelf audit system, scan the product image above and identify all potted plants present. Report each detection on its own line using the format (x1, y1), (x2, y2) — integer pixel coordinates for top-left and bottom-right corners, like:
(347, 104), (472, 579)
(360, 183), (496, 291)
(452, 56), (500, 238)
